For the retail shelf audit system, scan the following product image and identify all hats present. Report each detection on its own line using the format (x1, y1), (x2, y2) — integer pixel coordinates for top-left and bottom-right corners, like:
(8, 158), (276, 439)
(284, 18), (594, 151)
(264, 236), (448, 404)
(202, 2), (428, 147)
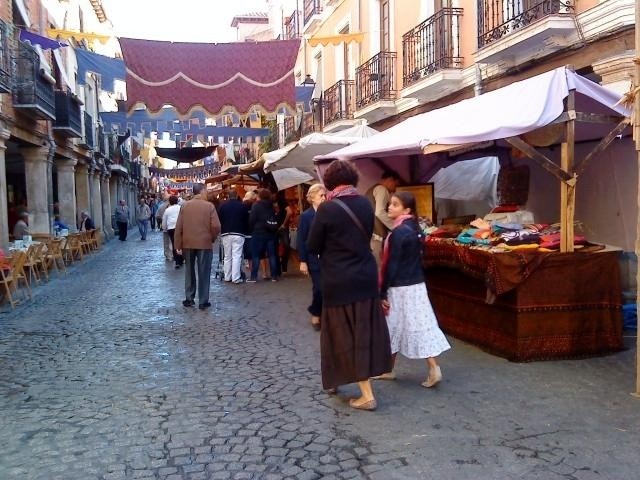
(253, 189), (272, 200)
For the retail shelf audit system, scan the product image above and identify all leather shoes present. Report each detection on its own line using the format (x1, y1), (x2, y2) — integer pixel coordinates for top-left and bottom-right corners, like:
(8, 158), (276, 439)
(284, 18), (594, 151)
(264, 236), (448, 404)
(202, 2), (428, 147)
(310, 317), (322, 332)
(199, 302), (211, 310)
(182, 300), (196, 306)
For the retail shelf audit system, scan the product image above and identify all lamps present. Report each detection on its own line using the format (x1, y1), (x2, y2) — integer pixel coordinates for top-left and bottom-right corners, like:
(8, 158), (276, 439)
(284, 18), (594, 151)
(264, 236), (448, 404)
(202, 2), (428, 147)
(299, 75), (320, 109)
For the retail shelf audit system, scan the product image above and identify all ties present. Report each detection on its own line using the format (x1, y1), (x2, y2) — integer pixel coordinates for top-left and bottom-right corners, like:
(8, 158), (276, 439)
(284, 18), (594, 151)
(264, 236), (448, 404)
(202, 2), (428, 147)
(122, 206), (123, 211)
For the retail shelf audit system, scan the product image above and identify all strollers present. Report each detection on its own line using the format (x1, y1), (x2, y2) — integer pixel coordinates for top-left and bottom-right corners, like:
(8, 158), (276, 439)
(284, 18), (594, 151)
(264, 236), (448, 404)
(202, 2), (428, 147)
(215, 241), (246, 283)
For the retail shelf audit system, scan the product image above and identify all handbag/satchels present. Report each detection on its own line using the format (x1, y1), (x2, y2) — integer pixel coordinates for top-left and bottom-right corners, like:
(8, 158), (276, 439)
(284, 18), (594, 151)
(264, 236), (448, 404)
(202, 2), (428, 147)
(277, 235), (285, 257)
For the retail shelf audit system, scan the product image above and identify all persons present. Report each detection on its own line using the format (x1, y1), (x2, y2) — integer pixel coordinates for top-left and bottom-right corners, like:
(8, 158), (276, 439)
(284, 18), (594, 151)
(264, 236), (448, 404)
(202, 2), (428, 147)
(13, 212), (30, 239)
(79, 210), (96, 250)
(111, 182), (300, 311)
(364, 167), (400, 274)
(306, 160), (393, 411)
(376, 190), (453, 388)
(53, 214), (69, 235)
(297, 184), (326, 332)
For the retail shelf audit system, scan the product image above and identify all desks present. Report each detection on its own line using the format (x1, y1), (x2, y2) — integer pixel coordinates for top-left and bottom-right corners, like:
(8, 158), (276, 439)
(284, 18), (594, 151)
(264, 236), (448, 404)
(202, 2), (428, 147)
(419, 227), (625, 362)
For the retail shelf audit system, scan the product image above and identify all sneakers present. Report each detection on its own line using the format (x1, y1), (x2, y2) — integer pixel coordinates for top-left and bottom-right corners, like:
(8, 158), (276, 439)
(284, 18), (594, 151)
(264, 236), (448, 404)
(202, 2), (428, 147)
(349, 397), (378, 412)
(421, 366), (443, 389)
(370, 370), (397, 381)
(233, 271), (287, 285)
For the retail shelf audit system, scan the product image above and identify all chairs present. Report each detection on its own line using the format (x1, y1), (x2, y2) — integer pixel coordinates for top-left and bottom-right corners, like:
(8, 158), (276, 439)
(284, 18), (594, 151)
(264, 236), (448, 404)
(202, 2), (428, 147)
(0, 226), (102, 311)
